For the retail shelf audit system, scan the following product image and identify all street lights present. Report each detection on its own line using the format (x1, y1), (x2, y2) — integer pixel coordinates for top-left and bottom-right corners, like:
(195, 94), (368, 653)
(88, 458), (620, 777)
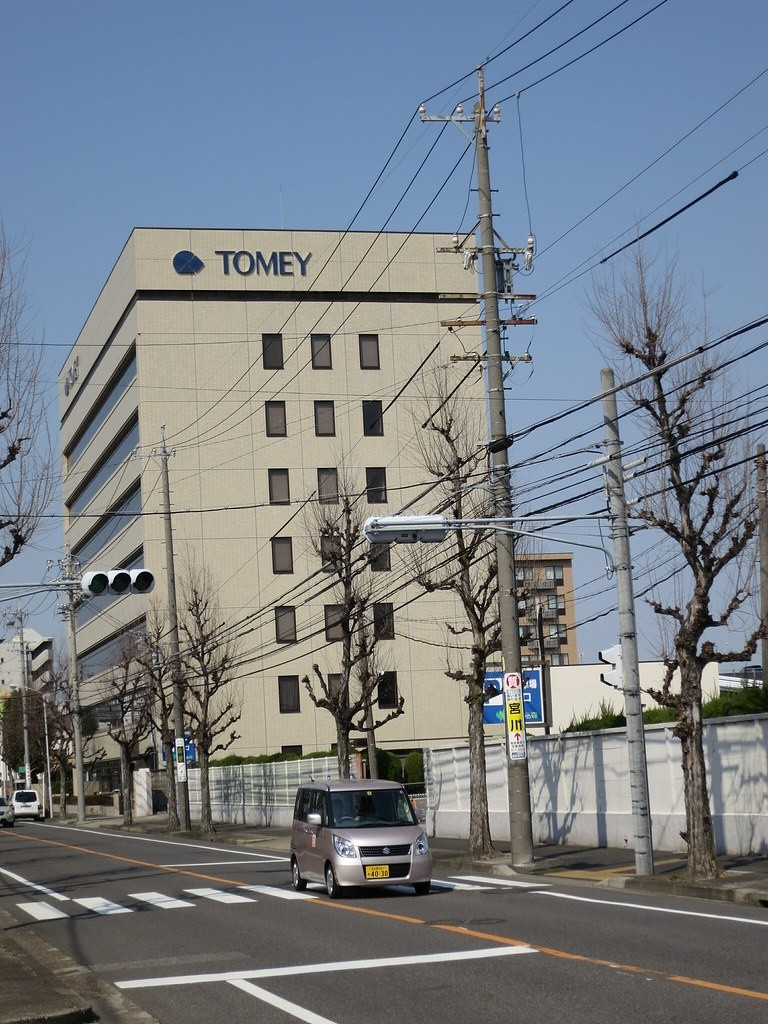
(7, 685), (53, 817)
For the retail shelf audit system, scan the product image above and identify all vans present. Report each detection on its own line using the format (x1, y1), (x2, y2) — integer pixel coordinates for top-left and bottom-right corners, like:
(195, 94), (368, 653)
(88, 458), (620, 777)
(8, 790), (41, 821)
(288, 778), (433, 899)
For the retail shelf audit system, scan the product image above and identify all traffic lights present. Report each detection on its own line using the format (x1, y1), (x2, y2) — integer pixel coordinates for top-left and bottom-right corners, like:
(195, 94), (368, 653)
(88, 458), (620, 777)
(598, 647), (621, 693)
(364, 514), (450, 545)
(82, 569), (155, 596)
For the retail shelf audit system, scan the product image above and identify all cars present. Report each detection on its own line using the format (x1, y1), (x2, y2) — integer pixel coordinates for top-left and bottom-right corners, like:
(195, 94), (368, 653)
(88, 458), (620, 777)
(0, 796), (15, 827)
